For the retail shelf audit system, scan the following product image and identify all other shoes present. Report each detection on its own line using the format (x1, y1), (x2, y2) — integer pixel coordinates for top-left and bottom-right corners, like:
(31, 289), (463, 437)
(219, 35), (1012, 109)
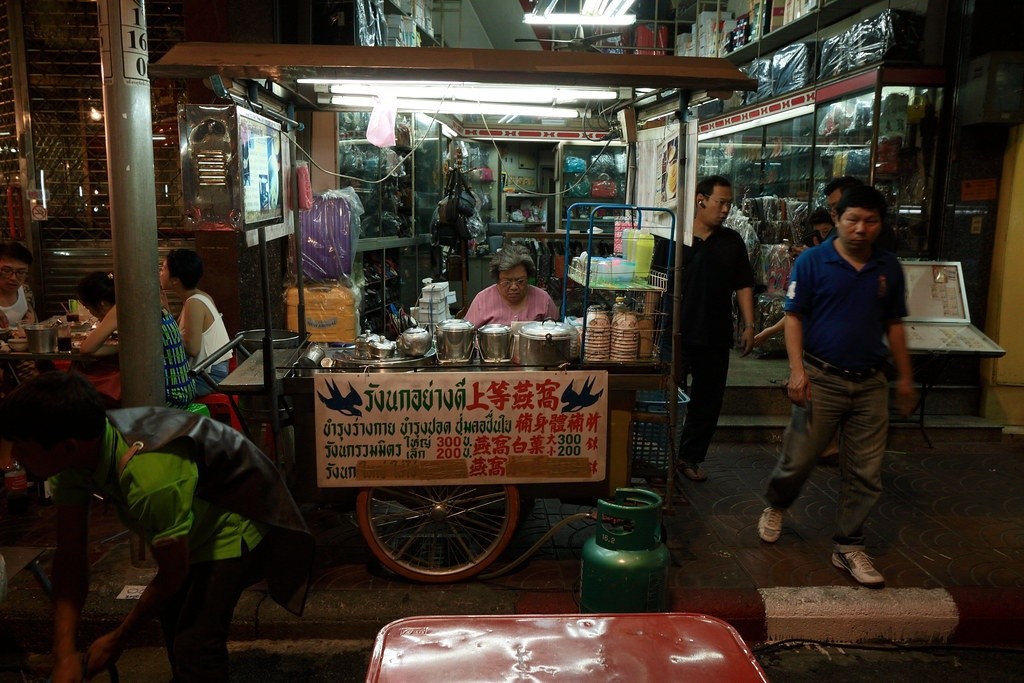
(360, 258), (413, 333)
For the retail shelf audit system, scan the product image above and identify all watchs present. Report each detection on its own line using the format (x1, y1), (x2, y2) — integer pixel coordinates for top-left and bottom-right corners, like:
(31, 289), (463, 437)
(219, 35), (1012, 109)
(744, 322), (756, 329)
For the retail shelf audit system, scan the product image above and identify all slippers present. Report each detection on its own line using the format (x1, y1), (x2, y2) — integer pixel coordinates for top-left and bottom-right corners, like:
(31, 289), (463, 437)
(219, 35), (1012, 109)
(679, 460), (707, 482)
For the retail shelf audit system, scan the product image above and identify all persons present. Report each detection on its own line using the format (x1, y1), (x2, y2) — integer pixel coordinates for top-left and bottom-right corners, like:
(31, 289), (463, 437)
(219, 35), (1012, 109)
(158, 248), (233, 396)
(0, 241), (34, 338)
(463, 243), (560, 327)
(651, 175), (756, 481)
(789, 176), (898, 469)
(77, 271), (196, 409)
(0, 372), (320, 683)
(758, 185), (916, 585)
(753, 205), (834, 345)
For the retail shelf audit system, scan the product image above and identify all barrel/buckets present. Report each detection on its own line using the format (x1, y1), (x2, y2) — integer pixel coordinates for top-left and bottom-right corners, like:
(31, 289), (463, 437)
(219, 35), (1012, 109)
(476, 324), (516, 365)
(23, 324), (58, 354)
(435, 320), (477, 365)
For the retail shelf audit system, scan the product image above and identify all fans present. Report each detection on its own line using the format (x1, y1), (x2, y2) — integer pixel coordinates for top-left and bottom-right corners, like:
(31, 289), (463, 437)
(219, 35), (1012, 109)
(513, 0), (623, 53)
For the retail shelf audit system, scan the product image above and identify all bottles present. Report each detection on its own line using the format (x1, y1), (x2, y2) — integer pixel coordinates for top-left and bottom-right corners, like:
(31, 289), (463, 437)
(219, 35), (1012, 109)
(611, 296), (626, 312)
(622, 228), (654, 277)
(5, 457), (27, 514)
(639, 316), (654, 358)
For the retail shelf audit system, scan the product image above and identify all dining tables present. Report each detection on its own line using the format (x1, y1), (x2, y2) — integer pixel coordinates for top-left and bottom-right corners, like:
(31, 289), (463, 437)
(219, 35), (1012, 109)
(0, 342), (122, 391)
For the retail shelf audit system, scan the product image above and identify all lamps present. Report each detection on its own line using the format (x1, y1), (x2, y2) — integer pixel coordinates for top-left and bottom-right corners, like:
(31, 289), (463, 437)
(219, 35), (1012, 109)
(603, 0), (623, 16)
(616, 0), (635, 16)
(641, 91), (719, 126)
(313, 75), (633, 103)
(315, 96), (594, 124)
(589, 0), (602, 15)
(530, 0), (558, 19)
(521, 12), (637, 26)
(582, 0), (595, 14)
(595, 0), (610, 15)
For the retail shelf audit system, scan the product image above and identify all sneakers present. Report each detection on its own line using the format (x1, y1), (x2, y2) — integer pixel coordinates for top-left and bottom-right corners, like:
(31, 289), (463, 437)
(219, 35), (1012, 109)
(832, 549), (885, 584)
(757, 507), (785, 542)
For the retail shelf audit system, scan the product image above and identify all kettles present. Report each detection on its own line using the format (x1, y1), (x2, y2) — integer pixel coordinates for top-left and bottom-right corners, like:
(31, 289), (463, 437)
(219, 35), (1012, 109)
(398, 323), (432, 356)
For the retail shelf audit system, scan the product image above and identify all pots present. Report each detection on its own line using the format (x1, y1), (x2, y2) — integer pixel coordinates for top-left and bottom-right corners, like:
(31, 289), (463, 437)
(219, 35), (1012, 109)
(509, 318), (572, 365)
(331, 346), (433, 372)
(235, 328), (302, 367)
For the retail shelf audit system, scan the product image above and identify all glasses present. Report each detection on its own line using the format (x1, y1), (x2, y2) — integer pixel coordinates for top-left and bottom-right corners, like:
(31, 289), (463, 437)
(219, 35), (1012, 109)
(825, 205), (840, 215)
(497, 276), (529, 288)
(705, 193), (732, 207)
(0, 270), (28, 280)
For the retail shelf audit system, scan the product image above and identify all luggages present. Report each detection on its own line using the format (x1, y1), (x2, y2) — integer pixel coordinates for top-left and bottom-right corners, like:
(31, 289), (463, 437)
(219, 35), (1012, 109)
(288, 284), (358, 343)
(287, 191), (352, 285)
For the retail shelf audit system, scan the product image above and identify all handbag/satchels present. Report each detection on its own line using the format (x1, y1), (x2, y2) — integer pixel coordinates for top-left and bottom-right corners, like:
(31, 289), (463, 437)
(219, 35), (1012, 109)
(566, 173), (591, 197)
(590, 154), (615, 172)
(561, 203), (626, 220)
(431, 155), (495, 282)
(563, 156), (586, 172)
(591, 173), (617, 199)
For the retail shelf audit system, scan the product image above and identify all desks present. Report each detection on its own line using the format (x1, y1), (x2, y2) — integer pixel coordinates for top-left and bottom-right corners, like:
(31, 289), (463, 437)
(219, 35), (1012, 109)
(360, 606), (774, 683)
(0, 539), (124, 683)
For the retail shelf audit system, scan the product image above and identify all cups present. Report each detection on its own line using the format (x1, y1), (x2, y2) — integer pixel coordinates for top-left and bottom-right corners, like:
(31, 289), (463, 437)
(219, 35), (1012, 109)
(65, 314), (80, 326)
(572, 256), (635, 288)
(56, 329), (73, 354)
(16, 320), (32, 338)
(298, 340), (326, 367)
(356, 329), (395, 359)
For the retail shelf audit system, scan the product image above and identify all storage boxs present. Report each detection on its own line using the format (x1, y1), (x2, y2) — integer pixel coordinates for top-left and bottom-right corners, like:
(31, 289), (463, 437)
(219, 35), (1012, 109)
(425, 18), (431, 27)
(722, 19), (737, 31)
(737, 12), (748, 27)
(414, 0), (424, 9)
(426, 26), (435, 37)
(754, 2), (767, 15)
(801, 0), (808, 16)
(414, 2), (425, 27)
(749, 10), (755, 24)
(393, 0), (401, 8)
(685, 47), (696, 57)
(385, 14), (404, 26)
(794, 0), (801, 19)
(404, 32), (416, 47)
(388, 26), (403, 41)
(691, 24), (697, 34)
(713, 21), (722, 31)
(771, 38), (823, 94)
(783, 0), (794, 24)
(733, 35), (748, 47)
(769, 0), (784, 33)
(698, 36), (706, 47)
(746, 59), (768, 106)
(402, 0), (411, 13)
(691, 35), (698, 46)
(698, 25), (706, 35)
(677, 34), (690, 44)
(720, 32), (727, 40)
(632, 383), (691, 480)
(387, 37), (401, 47)
(734, 26), (748, 39)
(818, 8), (923, 77)
(698, 46), (707, 57)
(748, 0), (754, 9)
(753, 13), (766, 27)
(403, 18), (417, 33)
(751, 27), (764, 40)
(697, 11), (732, 27)
(425, 7), (432, 18)
(752, 0), (760, 5)
(426, 0), (433, 9)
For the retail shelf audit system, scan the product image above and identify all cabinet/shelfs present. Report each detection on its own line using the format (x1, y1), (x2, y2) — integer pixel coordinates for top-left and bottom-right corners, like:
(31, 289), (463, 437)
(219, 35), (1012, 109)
(308, 0), (965, 362)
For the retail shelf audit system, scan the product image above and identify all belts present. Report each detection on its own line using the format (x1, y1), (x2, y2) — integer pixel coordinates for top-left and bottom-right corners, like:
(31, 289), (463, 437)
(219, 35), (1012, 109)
(801, 353), (870, 383)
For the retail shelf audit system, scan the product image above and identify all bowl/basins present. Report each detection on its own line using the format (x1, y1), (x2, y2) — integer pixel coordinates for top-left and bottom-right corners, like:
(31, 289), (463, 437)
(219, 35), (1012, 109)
(8, 338), (29, 352)
(584, 312), (638, 362)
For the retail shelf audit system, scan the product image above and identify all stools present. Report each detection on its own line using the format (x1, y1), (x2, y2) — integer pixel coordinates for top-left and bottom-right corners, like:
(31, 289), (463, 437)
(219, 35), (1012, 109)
(199, 391), (287, 464)
(186, 401), (216, 419)
